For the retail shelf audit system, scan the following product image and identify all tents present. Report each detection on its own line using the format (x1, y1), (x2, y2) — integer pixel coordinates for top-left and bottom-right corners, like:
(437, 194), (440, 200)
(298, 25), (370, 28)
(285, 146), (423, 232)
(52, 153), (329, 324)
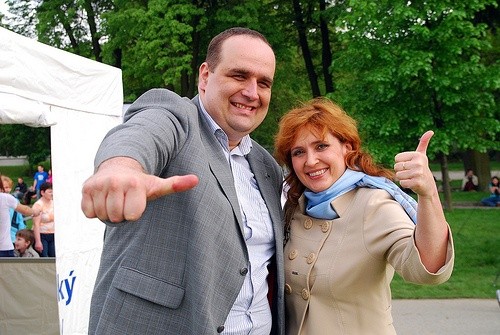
(1, 25), (124, 335)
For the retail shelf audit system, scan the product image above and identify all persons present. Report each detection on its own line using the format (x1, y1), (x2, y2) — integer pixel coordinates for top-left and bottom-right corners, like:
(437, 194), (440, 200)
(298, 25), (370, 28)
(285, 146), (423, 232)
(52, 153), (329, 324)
(274, 97), (456, 335)
(0, 164), (57, 259)
(81, 26), (287, 335)
(460, 168), (500, 207)
